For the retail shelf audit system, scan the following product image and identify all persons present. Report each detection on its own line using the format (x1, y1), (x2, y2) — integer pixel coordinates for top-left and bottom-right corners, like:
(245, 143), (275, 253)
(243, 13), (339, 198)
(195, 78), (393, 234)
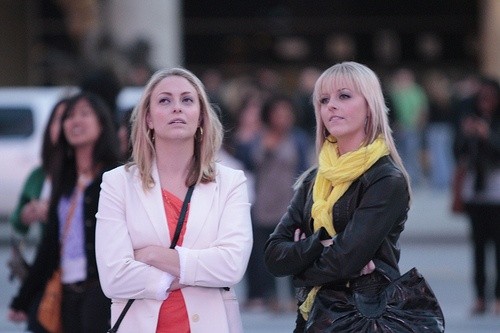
(262, 61), (410, 333)
(450, 78), (500, 317)
(197, 61), (325, 316)
(7, 93), (135, 333)
(384, 64), (456, 193)
(94, 68), (253, 333)
(24, 30), (157, 156)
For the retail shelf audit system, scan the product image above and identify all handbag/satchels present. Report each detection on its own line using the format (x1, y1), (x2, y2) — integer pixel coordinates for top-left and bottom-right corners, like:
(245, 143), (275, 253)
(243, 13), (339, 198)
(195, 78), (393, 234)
(303, 267), (446, 333)
(36, 271), (61, 333)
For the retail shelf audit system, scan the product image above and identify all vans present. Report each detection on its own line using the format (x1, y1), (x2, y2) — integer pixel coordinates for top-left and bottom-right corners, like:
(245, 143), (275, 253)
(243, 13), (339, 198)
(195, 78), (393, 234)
(0, 84), (85, 222)
